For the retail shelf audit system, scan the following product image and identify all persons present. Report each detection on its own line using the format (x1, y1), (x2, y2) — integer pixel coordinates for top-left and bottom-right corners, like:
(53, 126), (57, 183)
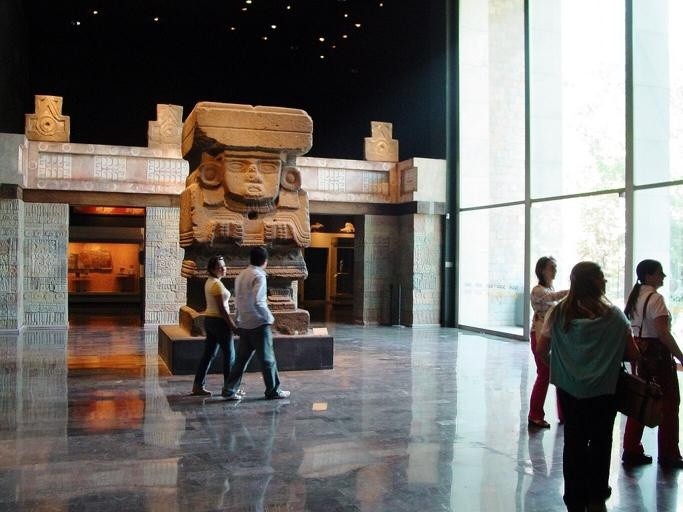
(191, 255), (249, 398)
(222, 398), (283, 512)
(172, 400), (235, 512)
(623, 257), (683, 470)
(178, 124), (312, 279)
(221, 245), (290, 400)
(613, 463), (679, 511)
(527, 256), (565, 429)
(523, 428), (569, 512)
(535, 260), (638, 512)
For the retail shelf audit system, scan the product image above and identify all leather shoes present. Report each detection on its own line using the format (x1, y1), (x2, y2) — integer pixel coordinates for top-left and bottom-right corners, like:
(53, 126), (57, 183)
(529, 419), (550, 428)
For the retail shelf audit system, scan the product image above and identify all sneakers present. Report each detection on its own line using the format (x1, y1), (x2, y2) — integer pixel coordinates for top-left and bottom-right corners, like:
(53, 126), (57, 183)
(657, 455), (683, 467)
(265, 390), (290, 398)
(220, 388), (246, 401)
(622, 452), (652, 464)
(194, 389), (212, 395)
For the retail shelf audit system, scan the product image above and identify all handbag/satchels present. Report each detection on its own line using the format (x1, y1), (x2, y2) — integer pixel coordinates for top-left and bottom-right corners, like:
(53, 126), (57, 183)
(634, 335), (673, 377)
(617, 351), (664, 428)
